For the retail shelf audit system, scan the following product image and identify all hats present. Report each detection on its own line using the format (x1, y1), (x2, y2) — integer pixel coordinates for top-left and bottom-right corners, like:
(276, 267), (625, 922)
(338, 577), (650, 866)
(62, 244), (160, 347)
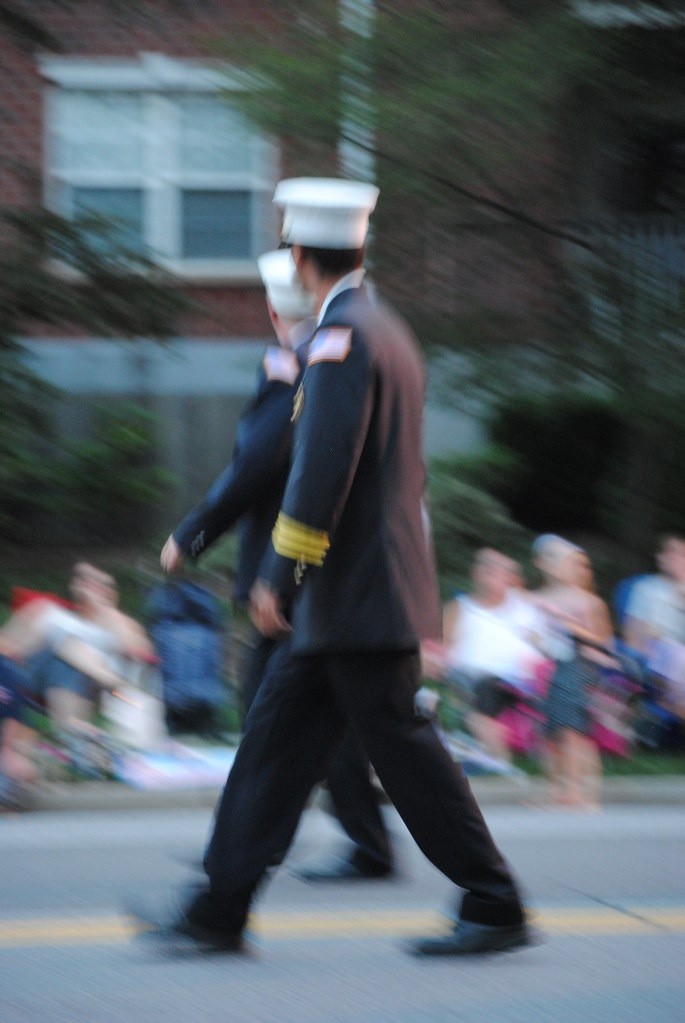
(271, 175), (380, 251)
(258, 248), (315, 319)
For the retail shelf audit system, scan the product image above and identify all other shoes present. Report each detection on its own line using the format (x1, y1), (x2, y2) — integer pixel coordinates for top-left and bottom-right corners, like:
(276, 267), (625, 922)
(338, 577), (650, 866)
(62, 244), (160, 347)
(125, 921), (249, 955)
(302, 858), (390, 883)
(401, 921), (530, 959)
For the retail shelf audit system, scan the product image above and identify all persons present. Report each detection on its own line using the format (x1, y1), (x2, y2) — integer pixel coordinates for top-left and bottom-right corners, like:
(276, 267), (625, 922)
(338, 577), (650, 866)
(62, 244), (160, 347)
(0, 533), (685, 812)
(125, 179), (538, 956)
